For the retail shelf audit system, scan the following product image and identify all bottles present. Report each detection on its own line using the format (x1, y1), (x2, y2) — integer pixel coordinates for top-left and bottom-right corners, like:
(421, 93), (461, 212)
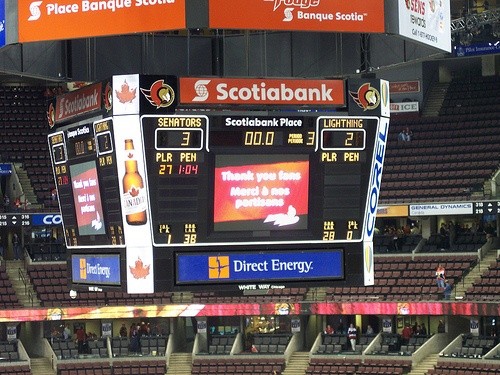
(122, 140), (148, 225)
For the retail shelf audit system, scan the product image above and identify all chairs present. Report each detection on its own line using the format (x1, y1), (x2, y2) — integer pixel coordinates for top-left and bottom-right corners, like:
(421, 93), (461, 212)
(0, 87), (500, 375)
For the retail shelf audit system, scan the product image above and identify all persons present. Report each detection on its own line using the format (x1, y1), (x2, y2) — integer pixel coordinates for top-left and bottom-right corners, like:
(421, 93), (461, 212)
(52, 322), (71, 339)
(2, 193), (32, 213)
(120, 323), (127, 337)
(411, 319), (444, 336)
(398, 127), (414, 142)
(347, 323), (357, 351)
(129, 321), (163, 336)
(402, 325), (413, 344)
(445, 220), (493, 241)
(76, 326), (92, 358)
(43, 86), (65, 98)
(129, 329), (143, 356)
(443, 280), (452, 301)
(440, 223), (446, 253)
(366, 325), (373, 336)
(0, 231), (7, 261)
(374, 223), (419, 252)
(435, 265), (446, 292)
(326, 325), (335, 335)
(11, 231), (20, 262)
